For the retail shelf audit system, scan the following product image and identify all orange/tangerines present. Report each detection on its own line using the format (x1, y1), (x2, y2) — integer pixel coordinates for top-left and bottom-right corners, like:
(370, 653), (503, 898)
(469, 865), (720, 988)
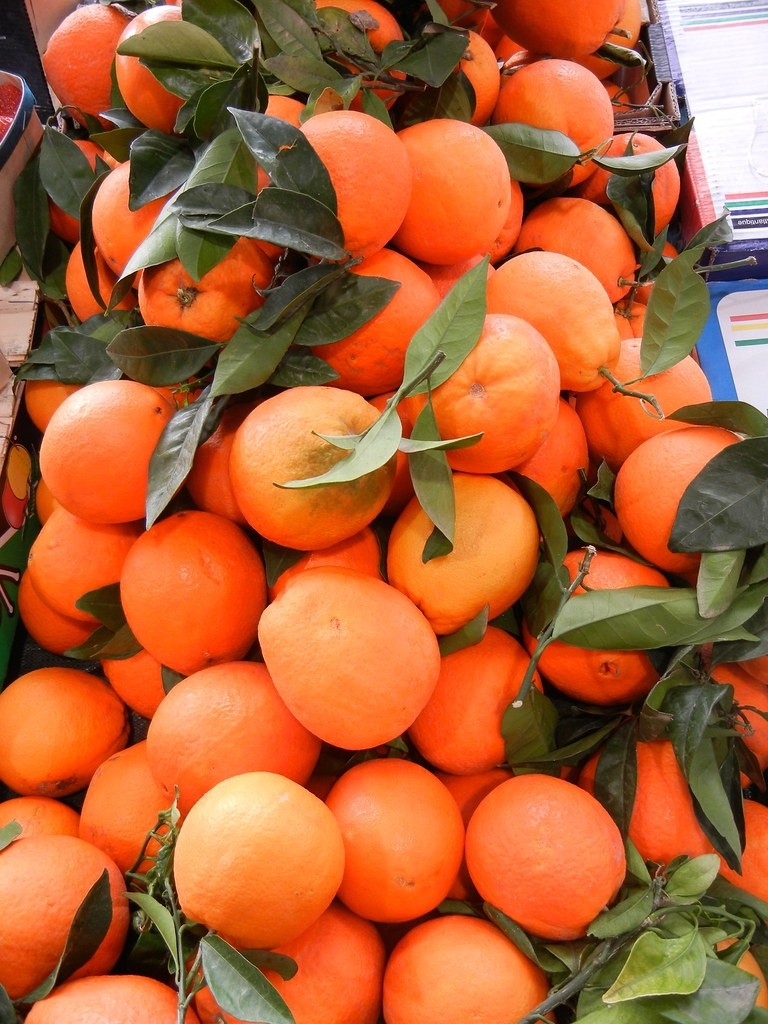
(0, 0), (768, 1024)
